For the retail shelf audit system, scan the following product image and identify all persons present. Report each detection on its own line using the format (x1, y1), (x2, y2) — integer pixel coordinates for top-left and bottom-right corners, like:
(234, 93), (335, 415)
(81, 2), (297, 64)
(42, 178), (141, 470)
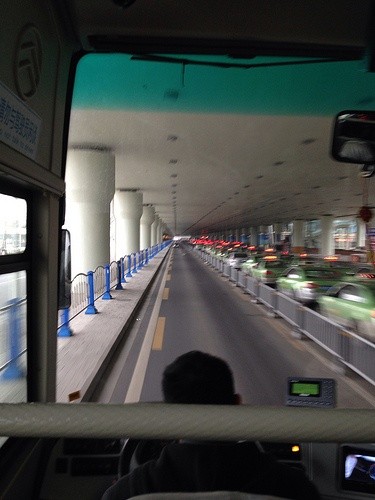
(100, 351), (321, 500)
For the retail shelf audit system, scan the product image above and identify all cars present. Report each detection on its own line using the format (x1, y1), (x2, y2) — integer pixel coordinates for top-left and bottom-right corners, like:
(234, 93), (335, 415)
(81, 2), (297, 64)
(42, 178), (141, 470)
(316, 272), (375, 342)
(183, 236), (375, 308)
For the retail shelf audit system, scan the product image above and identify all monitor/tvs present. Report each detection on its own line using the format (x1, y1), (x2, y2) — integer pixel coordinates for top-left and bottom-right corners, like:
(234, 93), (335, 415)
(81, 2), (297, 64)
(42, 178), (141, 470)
(338, 444), (375, 498)
